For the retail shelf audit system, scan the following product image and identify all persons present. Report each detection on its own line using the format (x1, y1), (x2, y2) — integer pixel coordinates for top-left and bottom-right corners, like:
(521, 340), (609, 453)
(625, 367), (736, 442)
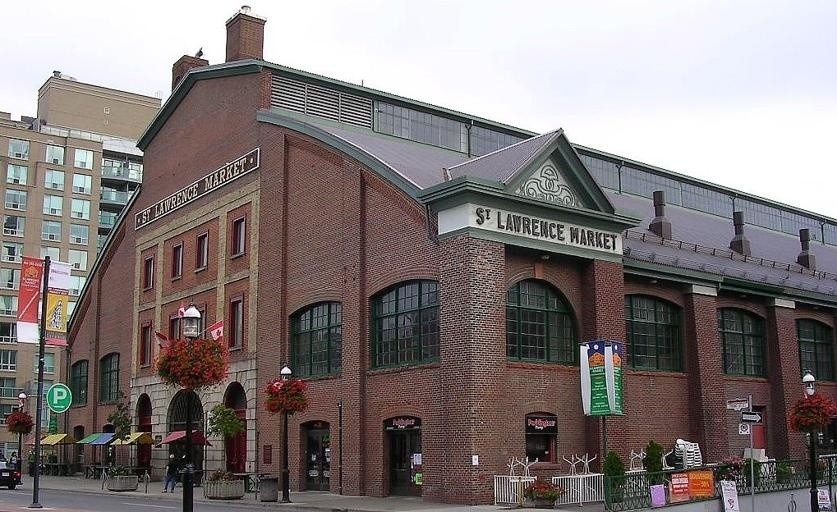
(8, 452), (18, 469)
(161, 454), (178, 493)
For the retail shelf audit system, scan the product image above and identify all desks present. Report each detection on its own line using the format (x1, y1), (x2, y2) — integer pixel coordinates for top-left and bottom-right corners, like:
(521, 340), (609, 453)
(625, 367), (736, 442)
(507, 449), (674, 502)
(45, 462), (272, 493)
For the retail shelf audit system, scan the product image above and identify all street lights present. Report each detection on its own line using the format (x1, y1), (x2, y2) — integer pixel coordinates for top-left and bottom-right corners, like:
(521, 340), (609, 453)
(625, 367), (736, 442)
(280, 364), (293, 503)
(802, 370), (819, 512)
(181, 298), (201, 512)
(18, 392), (27, 461)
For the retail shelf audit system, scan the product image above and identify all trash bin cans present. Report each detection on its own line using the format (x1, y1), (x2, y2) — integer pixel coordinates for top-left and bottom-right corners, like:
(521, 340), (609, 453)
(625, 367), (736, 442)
(261, 478), (278, 502)
(777, 461), (791, 483)
(28, 462), (36, 476)
(746, 459), (761, 486)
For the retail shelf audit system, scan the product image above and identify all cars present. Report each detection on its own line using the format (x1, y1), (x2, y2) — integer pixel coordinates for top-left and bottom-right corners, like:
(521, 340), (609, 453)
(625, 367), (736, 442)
(1, 462), (23, 489)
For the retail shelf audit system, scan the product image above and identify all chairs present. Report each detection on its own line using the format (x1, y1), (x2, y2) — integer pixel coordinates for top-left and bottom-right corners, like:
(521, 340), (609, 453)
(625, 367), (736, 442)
(768, 459), (776, 479)
(675, 439), (703, 469)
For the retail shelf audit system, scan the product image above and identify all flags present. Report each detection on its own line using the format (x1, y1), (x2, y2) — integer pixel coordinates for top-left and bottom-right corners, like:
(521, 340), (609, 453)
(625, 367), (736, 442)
(209, 321), (224, 346)
(15, 256), (71, 348)
(154, 332), (169, 350)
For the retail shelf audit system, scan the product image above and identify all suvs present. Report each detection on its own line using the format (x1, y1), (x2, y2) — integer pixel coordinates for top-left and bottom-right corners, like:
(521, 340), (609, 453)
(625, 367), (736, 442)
(29, 452), (57, 477)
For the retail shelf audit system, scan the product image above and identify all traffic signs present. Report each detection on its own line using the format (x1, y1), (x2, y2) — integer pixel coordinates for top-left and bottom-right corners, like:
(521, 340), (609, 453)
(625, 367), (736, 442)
(741, 412), (762, 424)
